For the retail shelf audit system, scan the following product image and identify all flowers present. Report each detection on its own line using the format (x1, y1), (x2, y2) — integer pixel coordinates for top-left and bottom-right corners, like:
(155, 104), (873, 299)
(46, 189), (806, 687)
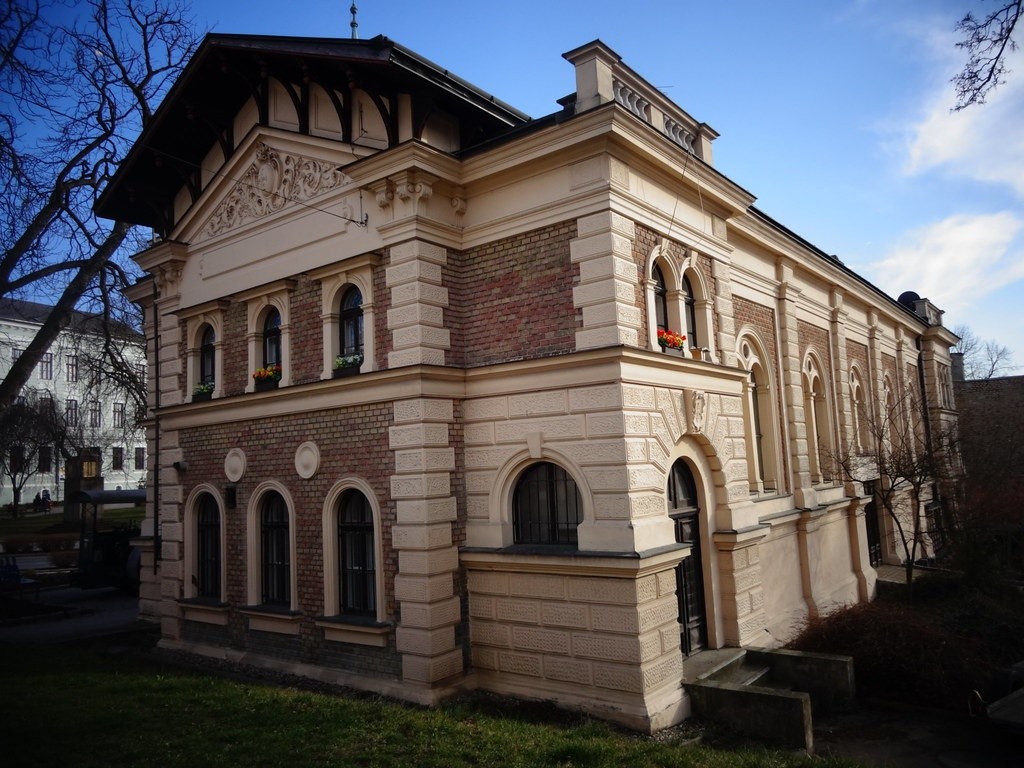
(252, 366), (280, 380)
(657, 329), (686, 351)
(192, 382), (214, 393)
(335, 355), (364, 367)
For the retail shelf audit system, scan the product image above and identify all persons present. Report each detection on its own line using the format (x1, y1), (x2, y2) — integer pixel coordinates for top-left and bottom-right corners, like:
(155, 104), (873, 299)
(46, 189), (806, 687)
(33, 491), (52, 515)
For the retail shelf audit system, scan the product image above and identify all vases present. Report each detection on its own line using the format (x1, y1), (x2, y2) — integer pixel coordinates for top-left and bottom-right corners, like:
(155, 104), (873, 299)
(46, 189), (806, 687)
(191, 394), (210, 402)
(332, 366), (359, 378)
(255, 381), (277, 392)
(662, 347), (683, 357)
